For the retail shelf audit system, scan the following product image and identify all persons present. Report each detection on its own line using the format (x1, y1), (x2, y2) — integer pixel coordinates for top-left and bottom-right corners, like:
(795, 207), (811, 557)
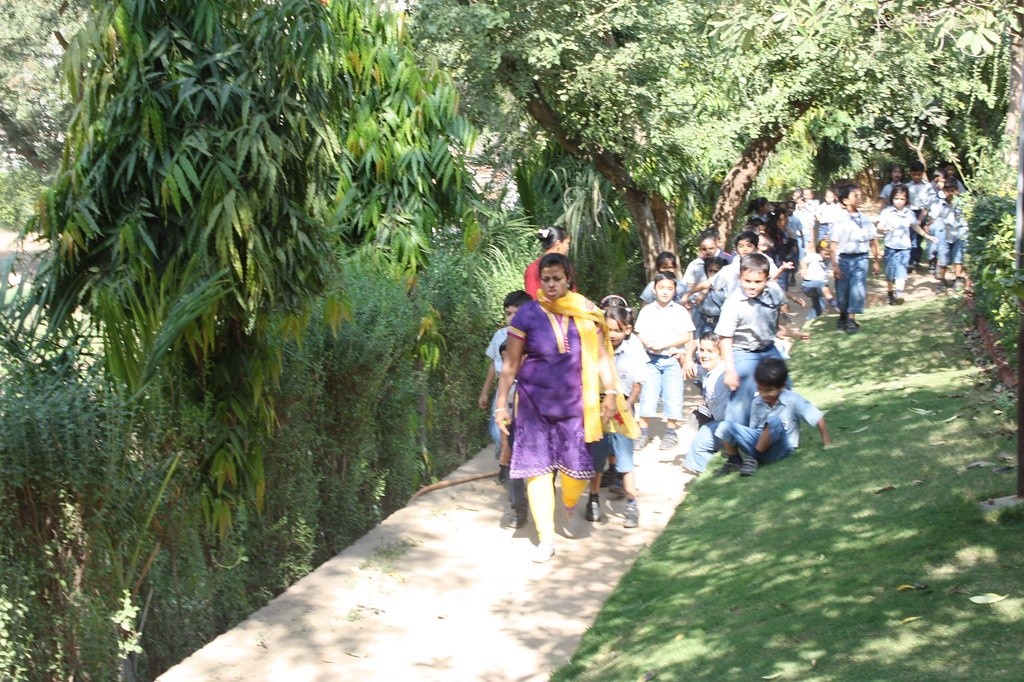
(478, 162), (975, 528)
(495, 252), (619, 562)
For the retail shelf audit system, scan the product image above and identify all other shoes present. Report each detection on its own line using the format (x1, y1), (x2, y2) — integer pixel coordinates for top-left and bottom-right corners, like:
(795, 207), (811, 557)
(532, 542), (556, 563)
(632, 433), (650, 451)
(658, 433), (679, 450)
(712, 456), (742, 477)
(609, 479), (629, 496)
(562, 507), (579, 532)
(738, 455), (759, 476)
(623, 502), (640, 528)
(498, 464), (508, 484)
(507, 505), (528, 528)
(788, 261), (973, 340)
(494, 447), (501, 459)
(585, 501), (601, 522)
(600, 470), (614, 487)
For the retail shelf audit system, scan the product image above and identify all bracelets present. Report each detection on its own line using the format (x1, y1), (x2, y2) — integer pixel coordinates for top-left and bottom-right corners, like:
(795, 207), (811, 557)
(604, 390), (618, 395)
(494, 408), (508, 413)
